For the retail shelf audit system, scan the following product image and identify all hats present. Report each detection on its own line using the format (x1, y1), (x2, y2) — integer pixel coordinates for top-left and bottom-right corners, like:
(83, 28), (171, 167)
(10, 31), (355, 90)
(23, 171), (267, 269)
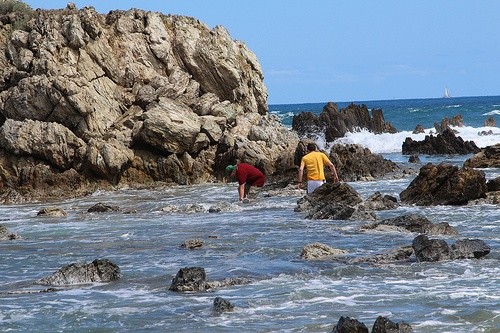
(226, 165), (237, 172)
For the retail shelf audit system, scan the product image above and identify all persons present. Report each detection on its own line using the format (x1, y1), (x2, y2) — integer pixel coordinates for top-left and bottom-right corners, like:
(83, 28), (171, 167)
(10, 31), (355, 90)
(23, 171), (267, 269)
(226, 163), (265, 201)
(298, 143), (339, 193)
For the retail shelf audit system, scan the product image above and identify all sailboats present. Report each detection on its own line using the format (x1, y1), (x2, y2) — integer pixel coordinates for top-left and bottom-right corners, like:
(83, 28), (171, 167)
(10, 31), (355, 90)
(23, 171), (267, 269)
(444, 87), (448, 98)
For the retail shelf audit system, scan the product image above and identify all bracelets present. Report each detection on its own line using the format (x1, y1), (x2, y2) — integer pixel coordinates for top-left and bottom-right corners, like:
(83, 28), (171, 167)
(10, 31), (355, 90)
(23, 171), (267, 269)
(298, 182), (302, 183)
